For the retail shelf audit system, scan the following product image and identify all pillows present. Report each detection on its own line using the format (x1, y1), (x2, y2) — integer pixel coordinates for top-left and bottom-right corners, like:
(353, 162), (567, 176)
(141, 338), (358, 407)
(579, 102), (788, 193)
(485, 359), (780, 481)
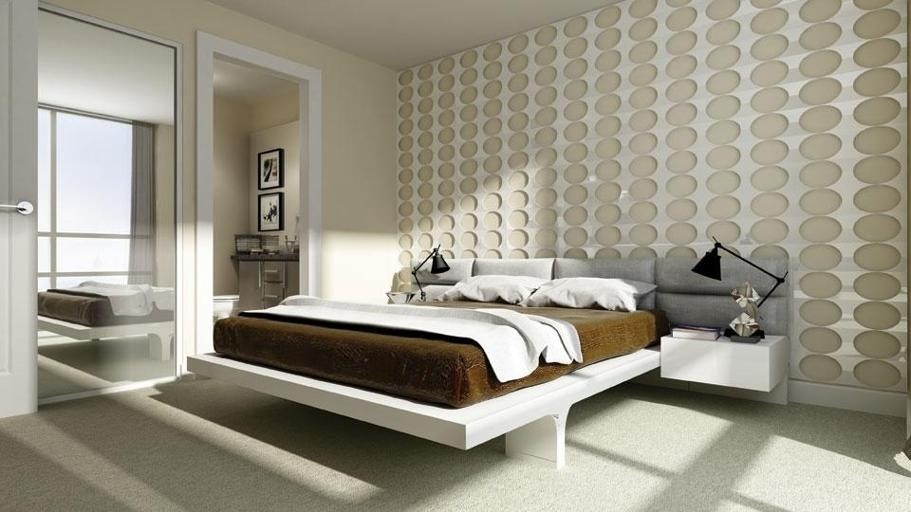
(434, 274), (658, 313)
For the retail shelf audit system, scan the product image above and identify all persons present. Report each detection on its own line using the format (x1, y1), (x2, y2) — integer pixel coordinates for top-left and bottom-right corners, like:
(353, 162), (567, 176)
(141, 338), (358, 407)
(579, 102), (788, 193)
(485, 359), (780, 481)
(267, 202), (277, 223)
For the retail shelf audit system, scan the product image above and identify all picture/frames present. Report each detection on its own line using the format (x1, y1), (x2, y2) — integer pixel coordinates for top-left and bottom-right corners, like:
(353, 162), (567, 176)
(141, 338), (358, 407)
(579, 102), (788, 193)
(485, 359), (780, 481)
(258, 149), (283, 231)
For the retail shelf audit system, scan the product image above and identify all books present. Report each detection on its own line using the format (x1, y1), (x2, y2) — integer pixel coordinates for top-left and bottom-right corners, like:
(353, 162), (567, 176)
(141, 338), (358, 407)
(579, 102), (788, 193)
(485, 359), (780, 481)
(672, 320), (722, 341)
(237, 234), (279, 255)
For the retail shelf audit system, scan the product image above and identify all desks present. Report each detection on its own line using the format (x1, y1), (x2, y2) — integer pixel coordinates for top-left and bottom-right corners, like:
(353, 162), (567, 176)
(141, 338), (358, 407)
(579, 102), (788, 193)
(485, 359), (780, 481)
(233, 254), (298, 291)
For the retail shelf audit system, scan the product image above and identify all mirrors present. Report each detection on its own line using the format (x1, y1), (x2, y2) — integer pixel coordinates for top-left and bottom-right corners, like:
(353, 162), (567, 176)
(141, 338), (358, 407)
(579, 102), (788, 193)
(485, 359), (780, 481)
(38, 9), (175, 400)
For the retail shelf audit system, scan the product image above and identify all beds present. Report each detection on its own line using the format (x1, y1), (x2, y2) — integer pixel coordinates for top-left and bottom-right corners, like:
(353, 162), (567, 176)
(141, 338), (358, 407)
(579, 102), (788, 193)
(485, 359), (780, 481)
(38, 282), (175, 363)
(188, 257), (656, 473)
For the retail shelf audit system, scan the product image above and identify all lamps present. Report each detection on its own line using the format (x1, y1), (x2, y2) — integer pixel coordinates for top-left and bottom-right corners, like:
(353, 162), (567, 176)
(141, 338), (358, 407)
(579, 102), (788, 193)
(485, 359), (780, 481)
(691, 241), (788, 339)
(412, 243), (450, 303)
